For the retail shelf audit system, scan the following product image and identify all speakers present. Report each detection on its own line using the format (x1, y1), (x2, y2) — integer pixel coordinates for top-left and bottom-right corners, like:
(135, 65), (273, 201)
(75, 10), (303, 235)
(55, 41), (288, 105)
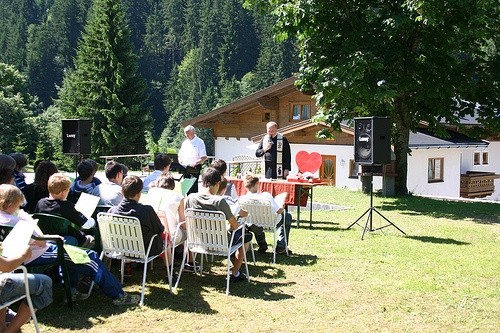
(354, 116), (391, 165)
(62, 119), (92, 155)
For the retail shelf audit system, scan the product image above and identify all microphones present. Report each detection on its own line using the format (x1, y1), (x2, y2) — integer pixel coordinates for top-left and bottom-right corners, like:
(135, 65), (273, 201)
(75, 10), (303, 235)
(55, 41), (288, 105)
(269, 135), (272, 142)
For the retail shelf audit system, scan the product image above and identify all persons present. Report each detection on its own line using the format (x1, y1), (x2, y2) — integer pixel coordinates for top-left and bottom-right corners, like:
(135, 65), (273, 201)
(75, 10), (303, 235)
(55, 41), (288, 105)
(35, 173), (103, 300)
(147, 174), (205, 272)
(91, 159), (125, 208)
(0, 153), (140, 305)
(255, 121), (291, 213)
(21, 160), (58, 215)
(68, 160), (104, 205)
(185, 168), (253, 282)
(144, 155), (171, 194)
(0, 242), (52, 333)
(177, 125), (207, 196)
(120, 166), (129, 180)
(8, 153), (29, 190)
(238, 174), (292, 254)
(107, 175), (177, 281)
(209, 157), (274, 251)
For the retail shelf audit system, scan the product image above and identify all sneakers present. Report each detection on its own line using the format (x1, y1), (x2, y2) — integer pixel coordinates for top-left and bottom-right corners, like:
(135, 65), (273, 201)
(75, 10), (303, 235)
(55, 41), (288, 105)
(112, 292), (140, 305)
(65, 292), (89, 302)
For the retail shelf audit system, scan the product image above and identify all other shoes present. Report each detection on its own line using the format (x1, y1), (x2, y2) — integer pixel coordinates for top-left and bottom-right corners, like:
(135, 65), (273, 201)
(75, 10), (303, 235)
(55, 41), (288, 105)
(119, 268), (131, 276)
(276, 246), (292, 254)
(80, 276), (95, 289)
(184, 262), (203, 272)
(164, 270), (178, 278)
(258, 247), (268, 253)
(230, 271), (246, 282)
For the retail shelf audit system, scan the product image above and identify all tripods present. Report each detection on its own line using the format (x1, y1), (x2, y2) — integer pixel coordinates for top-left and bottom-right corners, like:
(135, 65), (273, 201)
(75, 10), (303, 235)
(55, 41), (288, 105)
(346, 164), (406, 240)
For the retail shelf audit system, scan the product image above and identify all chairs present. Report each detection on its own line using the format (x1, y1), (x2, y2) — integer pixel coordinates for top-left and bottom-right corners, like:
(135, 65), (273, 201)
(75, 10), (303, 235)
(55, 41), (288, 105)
(0, 199), (289, 333)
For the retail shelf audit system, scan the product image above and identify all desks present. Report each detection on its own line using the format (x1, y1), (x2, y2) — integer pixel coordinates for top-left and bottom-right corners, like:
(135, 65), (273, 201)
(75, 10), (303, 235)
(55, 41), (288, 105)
(225, 176), (328, 229)
(100, 154), (151, 176)
(226, 160), (262, 177)
